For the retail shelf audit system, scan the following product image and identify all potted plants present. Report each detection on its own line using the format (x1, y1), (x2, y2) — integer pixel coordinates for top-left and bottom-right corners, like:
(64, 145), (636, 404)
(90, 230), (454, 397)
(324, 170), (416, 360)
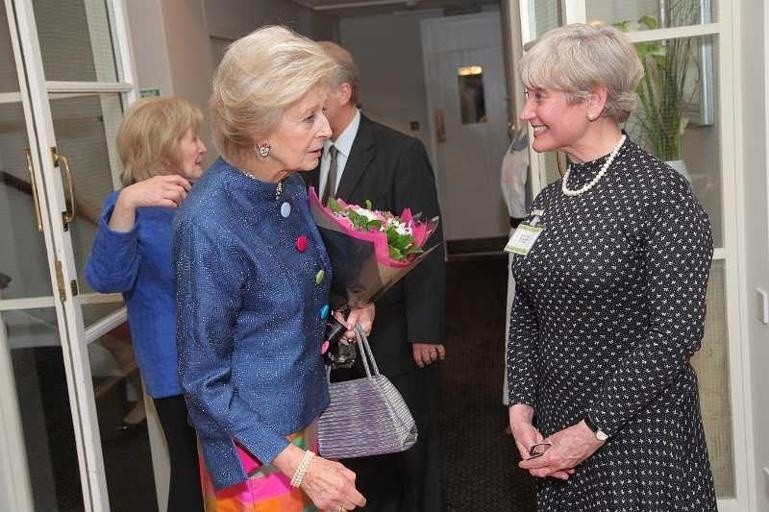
(613, 1), (699, 192)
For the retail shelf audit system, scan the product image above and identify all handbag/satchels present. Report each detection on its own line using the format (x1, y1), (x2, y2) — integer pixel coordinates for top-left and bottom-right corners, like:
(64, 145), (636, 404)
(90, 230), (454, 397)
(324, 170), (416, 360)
(318, 320), (418, 459)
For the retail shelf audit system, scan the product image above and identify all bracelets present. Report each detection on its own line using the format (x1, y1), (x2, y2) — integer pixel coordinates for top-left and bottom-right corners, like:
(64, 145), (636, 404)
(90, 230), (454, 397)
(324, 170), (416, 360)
(287, 449), (317, 489)
(584, 414), (612, 446)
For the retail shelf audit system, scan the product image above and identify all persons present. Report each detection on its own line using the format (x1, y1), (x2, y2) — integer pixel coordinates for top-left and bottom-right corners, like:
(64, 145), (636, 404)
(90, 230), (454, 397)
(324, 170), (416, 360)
(294, 36), (452, 512)
(170, 20), (385, 512)
(82, 96), (212, 511)
(502, 20), (722, 510)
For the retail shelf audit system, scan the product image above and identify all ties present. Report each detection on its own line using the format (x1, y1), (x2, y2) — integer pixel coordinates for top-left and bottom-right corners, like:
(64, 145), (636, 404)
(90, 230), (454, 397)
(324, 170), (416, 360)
(321, 145), (340, 207)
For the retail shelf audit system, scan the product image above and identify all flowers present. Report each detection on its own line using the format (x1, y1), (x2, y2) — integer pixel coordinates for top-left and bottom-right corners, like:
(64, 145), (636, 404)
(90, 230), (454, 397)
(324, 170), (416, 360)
(308, 184), (441, 369)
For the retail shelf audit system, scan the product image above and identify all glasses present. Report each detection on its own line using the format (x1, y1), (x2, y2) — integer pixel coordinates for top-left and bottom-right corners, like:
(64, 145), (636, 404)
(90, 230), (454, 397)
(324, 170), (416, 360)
(527, 443), (552, 461)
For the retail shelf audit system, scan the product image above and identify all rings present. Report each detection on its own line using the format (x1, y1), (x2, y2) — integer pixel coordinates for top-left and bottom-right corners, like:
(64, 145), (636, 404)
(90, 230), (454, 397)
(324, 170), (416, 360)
(340, 506), (342, 512)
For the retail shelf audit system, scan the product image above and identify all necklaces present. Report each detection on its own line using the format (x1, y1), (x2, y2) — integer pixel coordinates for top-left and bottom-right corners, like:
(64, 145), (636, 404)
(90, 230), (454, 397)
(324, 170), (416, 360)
(559, 131), (627, 199)
